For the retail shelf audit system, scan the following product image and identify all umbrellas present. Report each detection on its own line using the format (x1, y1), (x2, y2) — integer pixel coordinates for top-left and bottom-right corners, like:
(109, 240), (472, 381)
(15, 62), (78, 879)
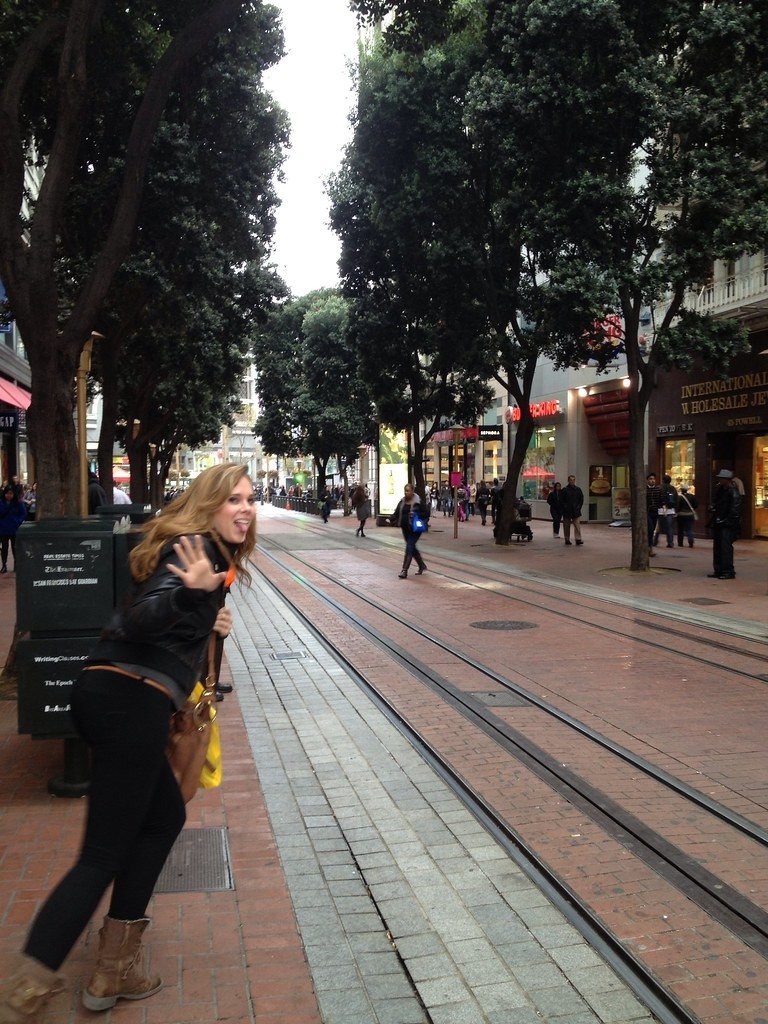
(523, 465), (555, 479)
(112, 465), (130, 483)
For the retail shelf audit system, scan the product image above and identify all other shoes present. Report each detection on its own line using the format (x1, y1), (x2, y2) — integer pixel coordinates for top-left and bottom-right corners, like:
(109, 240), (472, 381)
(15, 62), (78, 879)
(356, 529), (359, 536)
(553, 533), (560, 538)
(565, 540), (572, 545)
(361, 534), (366, 537)
(667, 542), (675, 548)
(576, 540), (583, 545)
(707, 572), (722, 578)
(0, 566), (7, 573)
(718, 574), (735, 580)
(649, 549), (656, 557)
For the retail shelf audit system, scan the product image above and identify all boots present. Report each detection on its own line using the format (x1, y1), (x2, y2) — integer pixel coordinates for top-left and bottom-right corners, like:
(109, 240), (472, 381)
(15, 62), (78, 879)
(81, 914), (164, 1010)
(413, 552), (427, 575)
(398, 556), (413, 578)
(0, 951), (66, 1024)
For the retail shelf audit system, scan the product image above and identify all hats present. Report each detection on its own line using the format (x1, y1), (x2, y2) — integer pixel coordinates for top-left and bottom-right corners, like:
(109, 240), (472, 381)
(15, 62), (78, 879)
(647, 472), (656, 479)
(680, 485), (687, 490)
(716, 469), (732, 478)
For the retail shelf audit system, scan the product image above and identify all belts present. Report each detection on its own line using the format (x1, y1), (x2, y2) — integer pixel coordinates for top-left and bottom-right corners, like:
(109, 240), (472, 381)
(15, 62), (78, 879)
(81, 665), (172, 697)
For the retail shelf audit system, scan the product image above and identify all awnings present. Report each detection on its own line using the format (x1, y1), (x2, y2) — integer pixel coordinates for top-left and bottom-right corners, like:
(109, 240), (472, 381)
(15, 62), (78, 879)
(0, 377), (33, 411)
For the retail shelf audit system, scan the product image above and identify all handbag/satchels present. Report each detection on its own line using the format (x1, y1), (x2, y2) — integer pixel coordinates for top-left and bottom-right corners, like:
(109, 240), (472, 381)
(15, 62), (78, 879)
(186, 681), (223, 792)
(164, 699), (212, 806)
(693, 510), (697, 520)
(412, 512), (425, 533)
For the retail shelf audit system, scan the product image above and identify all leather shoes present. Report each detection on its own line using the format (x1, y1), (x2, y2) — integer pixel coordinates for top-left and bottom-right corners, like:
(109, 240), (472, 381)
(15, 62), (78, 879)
(215, 691), (224, 701)
(215, 682), (232, 693)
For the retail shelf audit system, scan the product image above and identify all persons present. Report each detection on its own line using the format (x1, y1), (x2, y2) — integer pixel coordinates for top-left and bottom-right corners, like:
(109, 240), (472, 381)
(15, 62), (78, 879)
(254, 482), (312, 502)
(330, 481), (370, 506)
(112, 479), (133, 505)
(423, 477), (533, 542)
(0, 475), (37, 573)
(351, 486), (371, 537)
(320, 485), (331, 523)
(384, 484), (430, 578)
(707, 469), (741, 579)
(547, 482), (564, 538)
(87, 466), (107, 515)
(560, 475), (585, 546)
(645, 474), (698, 556)
(164, 486), (184, 502)
(0, 463), (256, 1024)
